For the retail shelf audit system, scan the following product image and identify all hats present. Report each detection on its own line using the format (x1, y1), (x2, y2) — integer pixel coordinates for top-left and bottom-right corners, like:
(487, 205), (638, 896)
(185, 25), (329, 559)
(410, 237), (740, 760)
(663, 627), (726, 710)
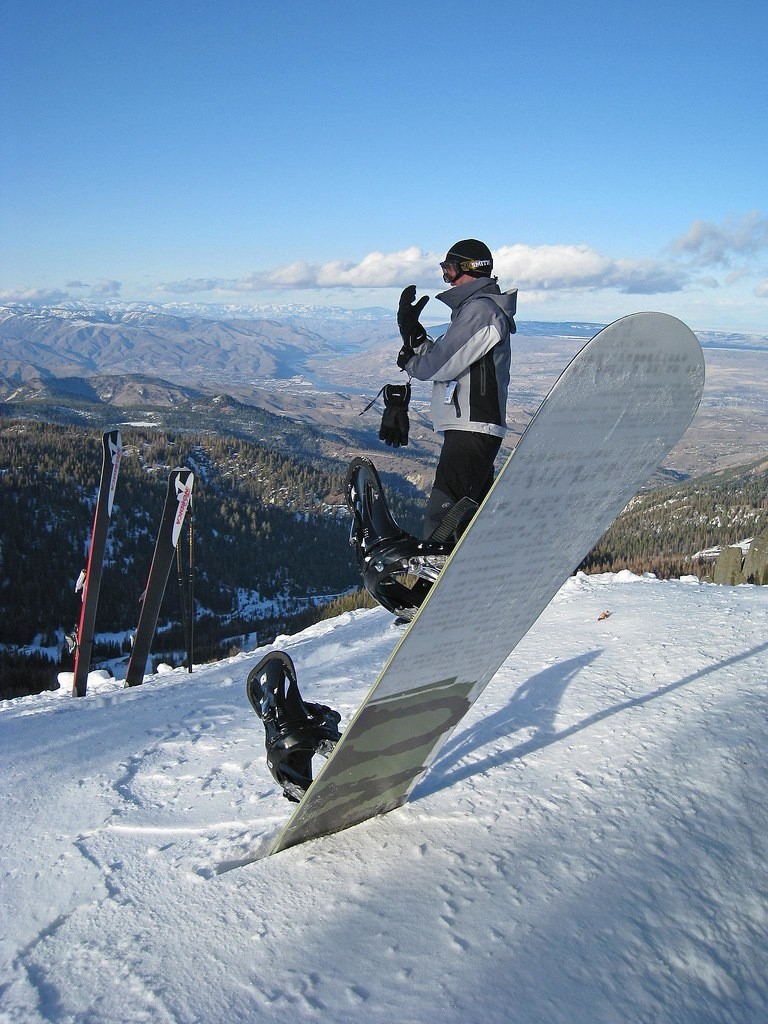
(446, 239), (493, 278)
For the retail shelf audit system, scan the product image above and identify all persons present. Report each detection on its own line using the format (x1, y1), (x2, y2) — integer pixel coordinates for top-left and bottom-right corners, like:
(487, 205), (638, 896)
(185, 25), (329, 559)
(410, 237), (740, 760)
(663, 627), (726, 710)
(396, 239), (517, 547)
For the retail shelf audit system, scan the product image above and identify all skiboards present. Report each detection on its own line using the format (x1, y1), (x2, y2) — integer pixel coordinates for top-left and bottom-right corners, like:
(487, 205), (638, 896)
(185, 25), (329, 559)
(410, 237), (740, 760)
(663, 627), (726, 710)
(69, 429), (197, 699)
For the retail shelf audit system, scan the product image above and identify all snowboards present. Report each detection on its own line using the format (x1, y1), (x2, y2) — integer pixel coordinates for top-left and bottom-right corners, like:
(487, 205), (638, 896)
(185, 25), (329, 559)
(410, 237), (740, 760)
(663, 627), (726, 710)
(266, 307), (710, 862)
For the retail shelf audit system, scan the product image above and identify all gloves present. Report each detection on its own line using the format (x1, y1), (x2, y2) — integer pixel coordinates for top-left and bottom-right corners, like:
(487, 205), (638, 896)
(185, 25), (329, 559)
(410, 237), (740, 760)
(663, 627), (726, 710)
(359, 384), (411, 448)
(397, 344), (415, 372)
(397, 285), (430, 348)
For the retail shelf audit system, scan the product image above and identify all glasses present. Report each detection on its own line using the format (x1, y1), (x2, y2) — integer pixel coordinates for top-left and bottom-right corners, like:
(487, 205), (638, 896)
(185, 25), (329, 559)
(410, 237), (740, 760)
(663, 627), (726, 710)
(439, 262), (464, 283)
